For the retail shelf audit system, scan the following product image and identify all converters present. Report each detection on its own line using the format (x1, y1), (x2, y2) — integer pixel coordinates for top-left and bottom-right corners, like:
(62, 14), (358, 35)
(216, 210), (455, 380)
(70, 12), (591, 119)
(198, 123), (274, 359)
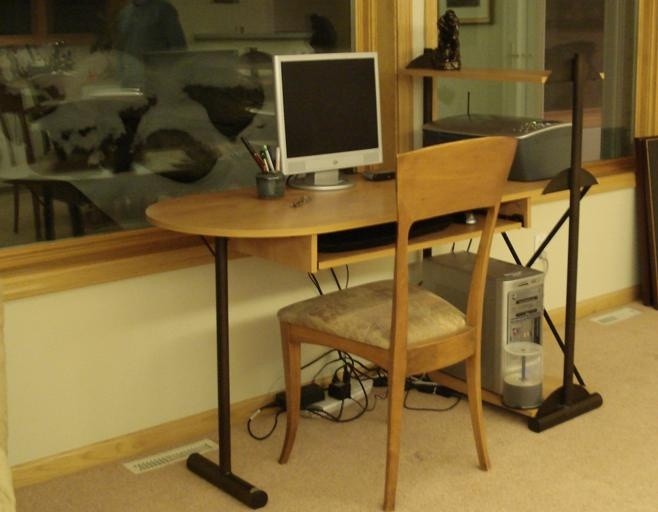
(329, 379), (353, 402)
(275, 382), (324, 409)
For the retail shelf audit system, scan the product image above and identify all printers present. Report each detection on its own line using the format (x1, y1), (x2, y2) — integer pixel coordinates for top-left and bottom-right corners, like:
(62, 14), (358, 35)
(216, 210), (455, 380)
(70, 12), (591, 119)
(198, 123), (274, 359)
(422, 110), (572, 181)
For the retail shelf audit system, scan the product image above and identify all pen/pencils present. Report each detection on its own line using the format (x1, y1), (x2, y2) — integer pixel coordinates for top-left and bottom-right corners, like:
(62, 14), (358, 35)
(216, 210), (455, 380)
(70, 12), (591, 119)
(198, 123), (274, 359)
(240, 136), (281, 173)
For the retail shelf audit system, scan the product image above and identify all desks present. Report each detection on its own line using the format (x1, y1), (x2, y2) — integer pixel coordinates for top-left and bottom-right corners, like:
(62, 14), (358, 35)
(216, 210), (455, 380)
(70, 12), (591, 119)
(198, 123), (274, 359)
(145, 41), (602, 512)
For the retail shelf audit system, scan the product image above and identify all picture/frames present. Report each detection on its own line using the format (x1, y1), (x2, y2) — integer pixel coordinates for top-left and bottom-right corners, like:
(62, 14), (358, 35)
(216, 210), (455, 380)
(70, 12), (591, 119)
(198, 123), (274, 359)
(435, 1), (492, 25)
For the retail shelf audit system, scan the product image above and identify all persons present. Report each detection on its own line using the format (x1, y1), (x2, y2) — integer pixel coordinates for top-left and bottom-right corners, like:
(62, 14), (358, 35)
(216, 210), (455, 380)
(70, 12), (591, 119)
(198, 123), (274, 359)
(108, 1), (189, 92)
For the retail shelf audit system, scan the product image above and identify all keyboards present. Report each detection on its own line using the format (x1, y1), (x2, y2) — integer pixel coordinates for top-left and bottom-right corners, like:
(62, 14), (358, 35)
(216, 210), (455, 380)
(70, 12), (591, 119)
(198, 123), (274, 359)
(317, 214), (465, 254)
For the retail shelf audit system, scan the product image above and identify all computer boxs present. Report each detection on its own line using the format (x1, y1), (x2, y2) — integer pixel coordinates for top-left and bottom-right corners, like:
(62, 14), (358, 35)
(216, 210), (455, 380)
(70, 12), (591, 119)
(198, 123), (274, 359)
(407, 251), (547, 397)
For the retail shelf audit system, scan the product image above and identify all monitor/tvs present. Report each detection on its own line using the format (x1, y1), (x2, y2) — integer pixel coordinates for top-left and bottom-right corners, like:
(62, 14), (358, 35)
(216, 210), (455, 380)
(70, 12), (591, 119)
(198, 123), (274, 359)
(273, 50), (383, 193)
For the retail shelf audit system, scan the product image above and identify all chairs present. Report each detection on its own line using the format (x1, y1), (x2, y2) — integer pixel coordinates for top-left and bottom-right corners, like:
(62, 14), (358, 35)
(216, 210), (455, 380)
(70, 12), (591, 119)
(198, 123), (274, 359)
(277, 135), (517, 512)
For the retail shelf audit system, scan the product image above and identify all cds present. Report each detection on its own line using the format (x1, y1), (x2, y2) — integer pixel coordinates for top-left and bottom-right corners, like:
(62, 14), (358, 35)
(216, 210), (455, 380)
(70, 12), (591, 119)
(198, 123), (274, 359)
(502, 374), (543, 408)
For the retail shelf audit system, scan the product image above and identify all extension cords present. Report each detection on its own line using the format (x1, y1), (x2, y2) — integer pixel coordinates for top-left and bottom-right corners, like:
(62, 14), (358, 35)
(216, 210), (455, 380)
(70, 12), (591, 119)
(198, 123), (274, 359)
(307, 376), (372, 417)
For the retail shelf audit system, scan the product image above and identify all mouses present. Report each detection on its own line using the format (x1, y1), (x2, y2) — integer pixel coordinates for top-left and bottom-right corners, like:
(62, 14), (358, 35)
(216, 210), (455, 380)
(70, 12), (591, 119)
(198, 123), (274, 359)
(466, 213), (477, 226)
